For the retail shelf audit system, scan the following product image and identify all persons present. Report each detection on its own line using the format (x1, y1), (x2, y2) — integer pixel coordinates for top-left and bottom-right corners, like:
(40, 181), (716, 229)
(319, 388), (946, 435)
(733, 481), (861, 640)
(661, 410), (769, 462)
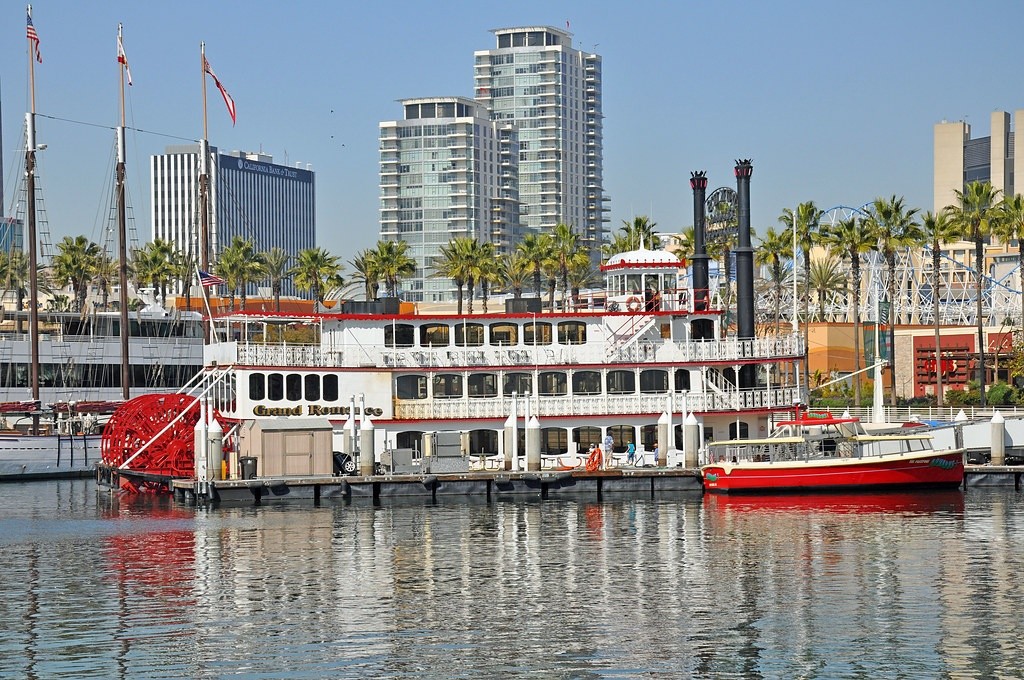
(624, 439), (635, 466)
(652, 443), (658, 467)
(586, 443), (596, 465)
(605, 430), (615, 468)
(710, 445), (769, 463)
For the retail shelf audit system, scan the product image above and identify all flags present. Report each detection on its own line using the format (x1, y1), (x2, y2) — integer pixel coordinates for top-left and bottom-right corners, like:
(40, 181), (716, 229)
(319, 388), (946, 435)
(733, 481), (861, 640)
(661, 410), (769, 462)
(197, 270), (230, 286)
(26, 12), (43, 65)
(116, 35), (133, 87)
(200, 45), (236, 129)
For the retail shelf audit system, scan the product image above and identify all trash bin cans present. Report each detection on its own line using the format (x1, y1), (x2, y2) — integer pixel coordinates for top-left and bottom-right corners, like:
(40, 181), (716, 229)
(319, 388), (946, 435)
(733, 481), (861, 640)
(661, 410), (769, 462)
(238, 456), (258, 480)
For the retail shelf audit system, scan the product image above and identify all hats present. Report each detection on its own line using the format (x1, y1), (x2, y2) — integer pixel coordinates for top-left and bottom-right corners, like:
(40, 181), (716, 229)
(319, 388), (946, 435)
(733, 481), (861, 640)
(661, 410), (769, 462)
(653, 443), (658, 447)
(627, 441), (631, 444)
(591, 443), (594, 446)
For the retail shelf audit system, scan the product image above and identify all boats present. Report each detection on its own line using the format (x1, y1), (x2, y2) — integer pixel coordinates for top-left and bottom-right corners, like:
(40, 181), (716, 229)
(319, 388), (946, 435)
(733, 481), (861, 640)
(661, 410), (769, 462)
(694, 400), (970, 495)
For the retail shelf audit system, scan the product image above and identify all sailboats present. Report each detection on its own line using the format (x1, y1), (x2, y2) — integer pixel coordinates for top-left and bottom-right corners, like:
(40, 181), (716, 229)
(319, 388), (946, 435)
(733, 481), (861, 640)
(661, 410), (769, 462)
(0, 110), (316, 480)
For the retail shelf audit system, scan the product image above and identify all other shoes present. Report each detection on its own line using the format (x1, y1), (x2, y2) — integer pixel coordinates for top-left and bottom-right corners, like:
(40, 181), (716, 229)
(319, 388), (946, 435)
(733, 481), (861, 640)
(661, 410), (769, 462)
(626, 461), (629, 464)
(630, 463), (633, 465)
